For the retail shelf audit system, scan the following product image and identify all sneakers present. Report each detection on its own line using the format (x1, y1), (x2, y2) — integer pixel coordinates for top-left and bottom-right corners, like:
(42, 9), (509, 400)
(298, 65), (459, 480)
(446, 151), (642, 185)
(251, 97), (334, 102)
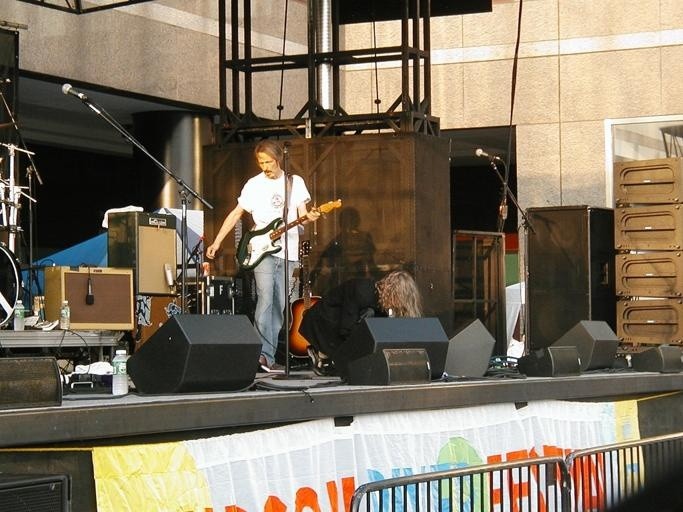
(270, 363), (286, 372)
(259, 355), (270, 372)
(307, 345), (329, 377)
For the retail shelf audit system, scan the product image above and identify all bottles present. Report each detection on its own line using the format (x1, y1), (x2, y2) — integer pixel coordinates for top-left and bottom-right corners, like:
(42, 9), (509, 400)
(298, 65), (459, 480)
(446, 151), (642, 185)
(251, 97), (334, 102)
(14, 300), (25, 331)
(112, 349), (128, 397)
(59, 299), (70, 331)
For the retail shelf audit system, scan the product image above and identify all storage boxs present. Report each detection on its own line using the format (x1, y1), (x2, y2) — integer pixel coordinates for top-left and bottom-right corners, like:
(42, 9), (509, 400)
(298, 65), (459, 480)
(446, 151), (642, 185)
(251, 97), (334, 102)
(612, 157), (683, 205)
(614, 251), (683, 298)
(616, 299), (683, 347)
(613, 207), (683, 251)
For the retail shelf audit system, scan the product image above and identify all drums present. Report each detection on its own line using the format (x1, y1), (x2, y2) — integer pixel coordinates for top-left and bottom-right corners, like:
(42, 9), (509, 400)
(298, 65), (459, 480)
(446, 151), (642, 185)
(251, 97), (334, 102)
(0, 242), (24, 329)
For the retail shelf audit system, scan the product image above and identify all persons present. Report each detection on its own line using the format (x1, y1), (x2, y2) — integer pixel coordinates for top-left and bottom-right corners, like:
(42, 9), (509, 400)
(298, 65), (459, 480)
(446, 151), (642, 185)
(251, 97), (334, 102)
(206, 142), (321, 368)
(298, 271), (422, 375)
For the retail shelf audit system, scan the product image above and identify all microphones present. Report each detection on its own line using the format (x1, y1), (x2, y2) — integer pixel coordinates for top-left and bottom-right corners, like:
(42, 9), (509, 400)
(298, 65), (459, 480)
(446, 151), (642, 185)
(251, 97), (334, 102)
(475, 148), (502, 161)
(282, 141), (292, 153)
(86, 279), (95, 303)
(62, 83), (88, 101)
(165, 264), (174, 289)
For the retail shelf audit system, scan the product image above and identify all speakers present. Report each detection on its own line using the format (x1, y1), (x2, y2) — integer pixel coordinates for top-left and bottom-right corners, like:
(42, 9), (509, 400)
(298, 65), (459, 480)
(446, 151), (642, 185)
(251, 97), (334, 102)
(444, 315), (498, 378)
(107, 212), (178, 296)
(527, 206), (617, 355)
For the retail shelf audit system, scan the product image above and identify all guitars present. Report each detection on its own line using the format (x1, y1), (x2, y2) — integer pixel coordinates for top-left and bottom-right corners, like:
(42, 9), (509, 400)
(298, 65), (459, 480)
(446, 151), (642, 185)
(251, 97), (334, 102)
(236, 199), (342, 271)
(288, 240), (323, 360)
